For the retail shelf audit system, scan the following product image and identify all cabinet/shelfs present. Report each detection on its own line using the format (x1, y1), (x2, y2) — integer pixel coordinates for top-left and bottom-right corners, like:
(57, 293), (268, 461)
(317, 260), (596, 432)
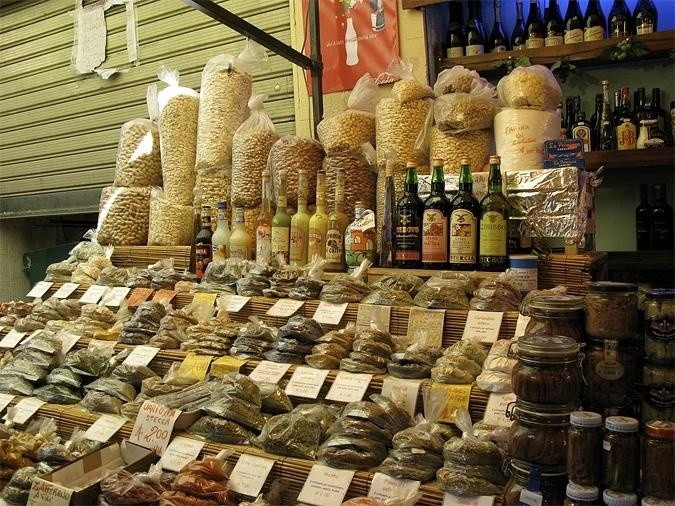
(402, 1), (674, 285)
(0, 167), (597, 504)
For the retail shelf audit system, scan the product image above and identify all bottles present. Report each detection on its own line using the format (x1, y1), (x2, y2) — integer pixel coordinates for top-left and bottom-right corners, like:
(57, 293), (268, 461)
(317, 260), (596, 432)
(510, 0), (524, 51)
(380, 148), (398, 268)
(343, 17), (359, 66)
(255, 170), (273, 271)
(211, 200), (232, 261)
(271, 170), (291, 268)
(369, 0), (386, 32)
(488, 0), (509, 53)
(558, 96), (593, 153)
(563, 0), (583, 45)
(544, 0), (563, 47)
(651, 183), (675, 250)
(420, 158), (450, 270)
(583, 0), (607, 42)
(524, 0), (544, 50)
(607, 0), (633, 38)
(448, 156), (479, 271)
(392, 159), (421, 269)
(443, 1), (463, 58)
(636, 184), (652, 251)
(463, 0), (486, 56)
(344, 200), (377, 275)
(188, 186), (204, 273)
(288, 169), (311, 266)
(592, 80), (675, 154)
(195, 206), (214, 284)
(307, 170), (328, 269)
(507, 256), (539, 297)
(633, 0), (658, 35)
(323, 168), (348, 272)
(478, 156), (510, 271)
(229, 204), (252, 262)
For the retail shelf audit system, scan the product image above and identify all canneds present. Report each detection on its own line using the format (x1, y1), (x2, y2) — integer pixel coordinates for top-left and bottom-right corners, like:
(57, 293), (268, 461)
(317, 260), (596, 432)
(640, 357), (675, 422)
(601, 490), (639, 505)
(568, 410), (603, 486)
(643, 290), (674, 368)
(585, 281), (641, 339)
(642, 493), (674, 506)
(564, 483), (599, 505)
(642, 419), (675, 499)
(602, 416), (639, 491)
(588, 340), (635, 407)
